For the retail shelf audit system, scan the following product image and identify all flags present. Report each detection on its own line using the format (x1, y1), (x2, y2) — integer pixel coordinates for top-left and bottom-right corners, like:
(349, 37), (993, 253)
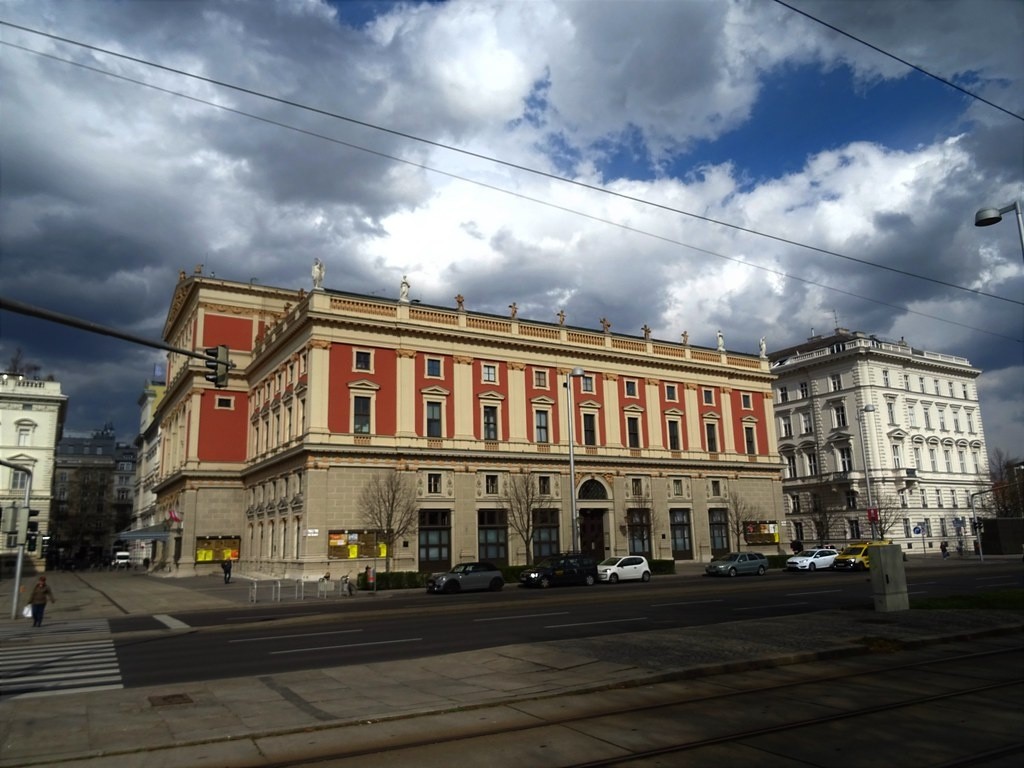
(169, 510), (181, 522)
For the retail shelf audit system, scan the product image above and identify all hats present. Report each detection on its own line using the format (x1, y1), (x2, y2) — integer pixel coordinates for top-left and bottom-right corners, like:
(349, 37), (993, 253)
(40, 576), (46, 581)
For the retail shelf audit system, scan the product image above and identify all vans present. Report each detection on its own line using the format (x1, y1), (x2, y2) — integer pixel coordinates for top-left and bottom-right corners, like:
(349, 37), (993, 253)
(111, 551), (131, 567)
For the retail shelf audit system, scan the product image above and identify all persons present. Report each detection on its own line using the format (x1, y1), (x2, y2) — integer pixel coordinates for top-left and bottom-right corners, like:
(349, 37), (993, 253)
(323, 572), (330, 580)
(641, 325), (651, 338)
(940, 542), (947, 560)
(681, 331), (689, 345)
(400, 275), (410, 299)
(223, 557), (231, 583)
(760, 336), (767, 357)
(557, 310), (565, 325)
(717, 331), (724, 349)
(366, 566), (374, 590)
(455, 294), (464, 310)
(509, 302), (518, 318)
(599, 318), (610, 331)
(143, 558), (149, 569)
(312, 258), (325, 287)
(28, 576), (54, 627)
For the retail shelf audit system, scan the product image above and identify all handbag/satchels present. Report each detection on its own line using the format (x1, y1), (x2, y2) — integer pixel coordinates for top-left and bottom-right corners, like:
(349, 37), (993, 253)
(23, 604), (32, 618)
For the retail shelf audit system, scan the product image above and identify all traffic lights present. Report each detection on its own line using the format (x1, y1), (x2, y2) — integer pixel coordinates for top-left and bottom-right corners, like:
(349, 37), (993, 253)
(17, 506), (39, 545)
(973, 521), (977, 530)
(978, 521), (983, 530)
(206, 345), (228, 388)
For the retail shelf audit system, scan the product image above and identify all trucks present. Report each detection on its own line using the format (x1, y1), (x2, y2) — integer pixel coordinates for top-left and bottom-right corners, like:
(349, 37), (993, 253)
(833, 541), (892, 570)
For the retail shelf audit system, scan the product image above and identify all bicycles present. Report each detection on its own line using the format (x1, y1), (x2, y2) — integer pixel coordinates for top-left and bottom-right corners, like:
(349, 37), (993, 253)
(340, 570), (357, 596)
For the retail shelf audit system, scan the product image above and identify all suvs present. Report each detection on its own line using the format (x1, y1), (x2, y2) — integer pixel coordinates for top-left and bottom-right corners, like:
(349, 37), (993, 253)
(519, 551), (599, 587)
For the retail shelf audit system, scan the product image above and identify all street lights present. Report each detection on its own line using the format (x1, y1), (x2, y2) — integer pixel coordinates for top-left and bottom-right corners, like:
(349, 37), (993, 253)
(563, 368), (585, 551)
(856, 405), (876, 539)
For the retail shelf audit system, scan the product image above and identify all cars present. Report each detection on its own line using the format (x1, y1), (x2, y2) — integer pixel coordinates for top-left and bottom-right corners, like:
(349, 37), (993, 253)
(705, 552), (769, 576)
(596, 556), (653, 584)
(426, 562), (504, 594)
(786, 549), (839, 571)
